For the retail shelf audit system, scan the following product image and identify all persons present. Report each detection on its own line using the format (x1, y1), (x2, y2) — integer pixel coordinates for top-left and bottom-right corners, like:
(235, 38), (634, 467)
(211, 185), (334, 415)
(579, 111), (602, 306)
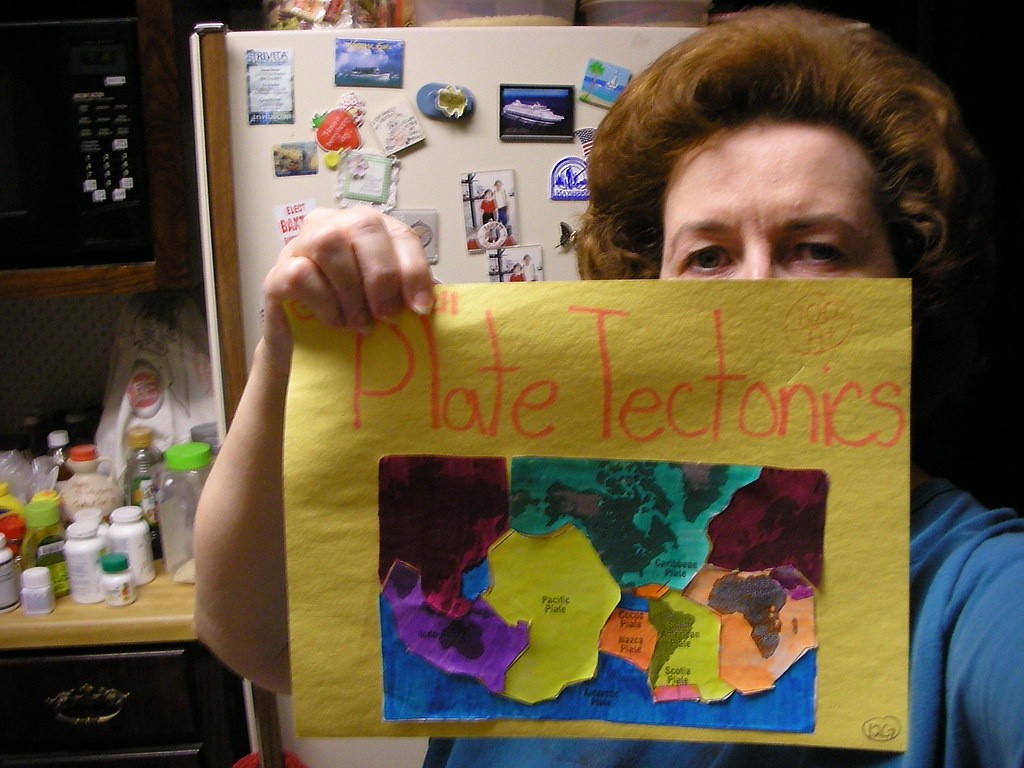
(194, 6), (1024, 768)
(510, 262), (524, 282)
(481, 189), (495, 225)
(494, 179), (510, 225)
(524, 255), (538, 282)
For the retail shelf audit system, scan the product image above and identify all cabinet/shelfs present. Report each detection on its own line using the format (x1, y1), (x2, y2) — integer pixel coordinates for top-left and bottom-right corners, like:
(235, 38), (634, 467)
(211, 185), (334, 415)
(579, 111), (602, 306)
(0, 639), (250, 768)
(0, 1), (202, 302)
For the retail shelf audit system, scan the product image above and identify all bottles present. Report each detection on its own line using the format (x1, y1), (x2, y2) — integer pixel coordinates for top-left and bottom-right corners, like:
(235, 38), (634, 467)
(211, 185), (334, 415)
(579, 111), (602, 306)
(20, 567), (56, 614)
(109, 505), (156, 585)
(0, 483), (27, 557)
(57, 445), (121, 526)
(0, 534), (20, 615)
(64, 523), (104, 603)
(47, 430), (75, 481)
(190, 422), (216, 446)
(124, 428), (165, 560)
(21, 503), (71, 599)
(73, 507), (110, 555)
(32, 490), (62, 520)
(158, 442), (216, 583)
(102, 555), (137, 605)
